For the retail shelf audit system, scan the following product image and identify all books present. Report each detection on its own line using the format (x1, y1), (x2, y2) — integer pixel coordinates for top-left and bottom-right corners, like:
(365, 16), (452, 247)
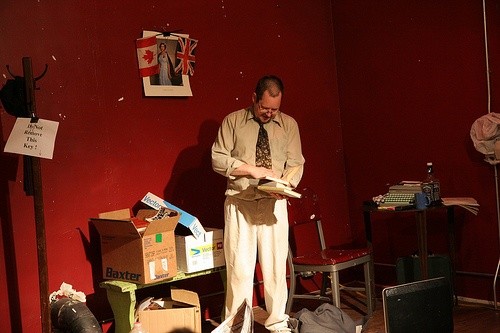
(441, 197), (480, 216)
(256, 176), (302, 198)
(378, 181), (423, 210)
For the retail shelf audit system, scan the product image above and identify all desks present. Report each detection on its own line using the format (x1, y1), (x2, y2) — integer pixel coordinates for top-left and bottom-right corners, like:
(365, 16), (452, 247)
(364, 196), (458, 280)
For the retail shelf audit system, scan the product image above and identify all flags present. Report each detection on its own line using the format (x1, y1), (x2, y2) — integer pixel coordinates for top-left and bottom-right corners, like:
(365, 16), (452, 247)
(135, 36), (160, 78)
(174, 36), (198, 76)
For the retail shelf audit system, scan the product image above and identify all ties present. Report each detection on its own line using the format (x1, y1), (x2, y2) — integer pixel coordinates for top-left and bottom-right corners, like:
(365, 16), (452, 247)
(253, 118), (273, 184)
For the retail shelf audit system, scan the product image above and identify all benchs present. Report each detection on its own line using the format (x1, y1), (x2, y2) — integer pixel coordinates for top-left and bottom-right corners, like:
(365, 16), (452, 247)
(98, 260), (227, 333)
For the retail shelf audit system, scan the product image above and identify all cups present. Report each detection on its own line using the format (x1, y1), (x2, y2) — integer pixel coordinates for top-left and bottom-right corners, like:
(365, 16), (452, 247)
(414, 192), (430, 210)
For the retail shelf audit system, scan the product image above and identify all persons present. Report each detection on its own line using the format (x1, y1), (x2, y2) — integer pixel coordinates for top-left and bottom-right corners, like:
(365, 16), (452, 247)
(157, 42), (176, 85)
(212, 76), (305, 333)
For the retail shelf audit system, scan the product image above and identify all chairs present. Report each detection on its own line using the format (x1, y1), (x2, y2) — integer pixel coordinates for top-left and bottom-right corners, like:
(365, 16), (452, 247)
(382, 277), (455, 333)
(286, 187), (373, 319)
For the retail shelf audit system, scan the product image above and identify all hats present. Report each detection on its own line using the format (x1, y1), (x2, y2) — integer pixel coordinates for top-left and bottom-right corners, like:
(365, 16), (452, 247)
(0, 75), (29, 118)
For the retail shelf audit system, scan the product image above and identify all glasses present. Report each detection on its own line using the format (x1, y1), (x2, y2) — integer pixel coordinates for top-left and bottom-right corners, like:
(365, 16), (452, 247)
(257, 97), (282, 116)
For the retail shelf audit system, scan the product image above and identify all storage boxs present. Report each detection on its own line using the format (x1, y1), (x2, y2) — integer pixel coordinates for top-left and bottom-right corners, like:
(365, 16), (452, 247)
(137, 287), (202, 333)
(88, 192), (225, 284)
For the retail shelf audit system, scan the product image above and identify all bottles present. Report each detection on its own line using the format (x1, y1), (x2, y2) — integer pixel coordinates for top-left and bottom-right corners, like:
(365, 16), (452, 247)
(423, 162), (441, 202)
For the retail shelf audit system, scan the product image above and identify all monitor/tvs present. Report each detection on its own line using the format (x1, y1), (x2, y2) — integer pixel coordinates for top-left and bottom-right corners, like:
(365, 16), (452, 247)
(383, 277), (454, 333)
